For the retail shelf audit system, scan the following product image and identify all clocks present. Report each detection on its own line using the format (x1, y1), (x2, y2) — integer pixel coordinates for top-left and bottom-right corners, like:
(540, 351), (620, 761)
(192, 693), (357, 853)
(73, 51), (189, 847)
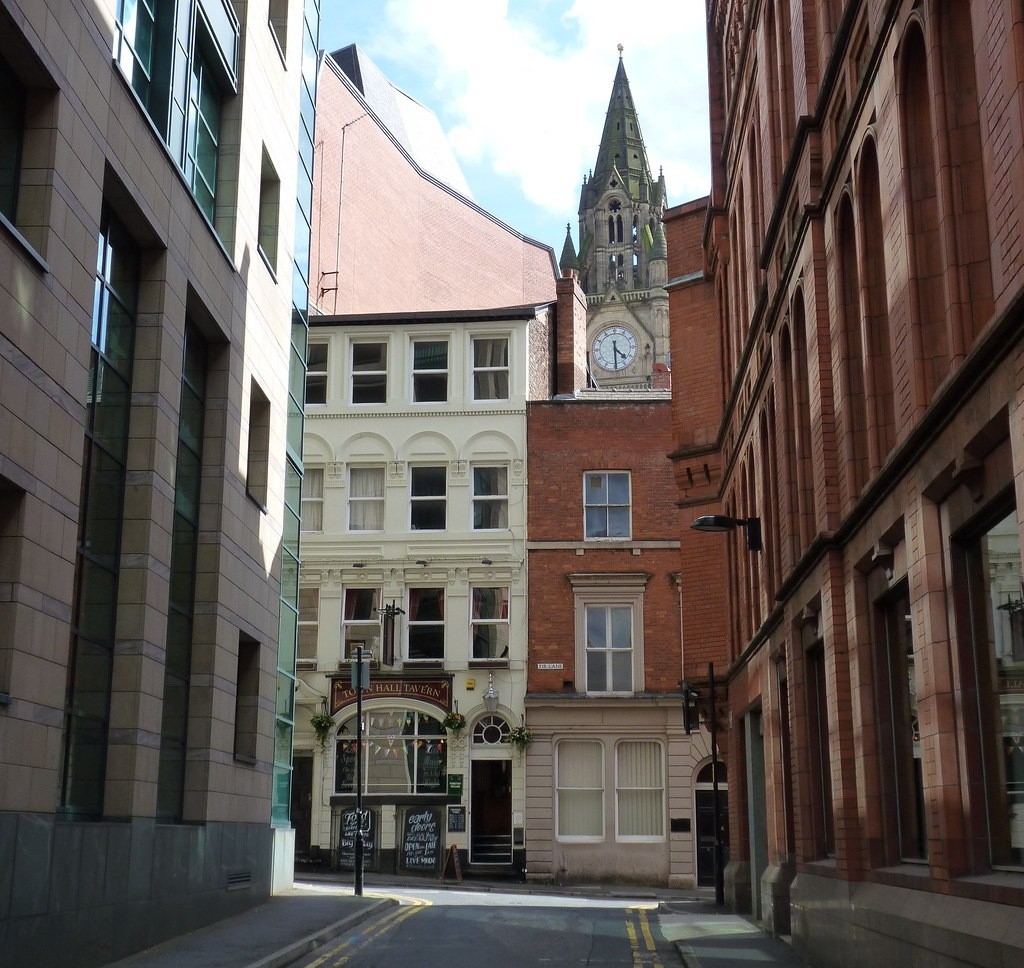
(592, 326), (638, 373)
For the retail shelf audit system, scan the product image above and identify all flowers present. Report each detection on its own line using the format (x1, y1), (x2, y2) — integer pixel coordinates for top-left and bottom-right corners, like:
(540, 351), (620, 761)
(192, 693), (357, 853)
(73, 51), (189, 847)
(508, 727), (533, 762)
(309, 715), (336, 748)
(440, 713), (468, 740)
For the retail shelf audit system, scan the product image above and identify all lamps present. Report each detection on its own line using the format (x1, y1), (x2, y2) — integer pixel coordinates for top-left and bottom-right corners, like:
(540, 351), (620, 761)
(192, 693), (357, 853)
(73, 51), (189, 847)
(482, 671), (498, 713)
(690, 515), (762, 551)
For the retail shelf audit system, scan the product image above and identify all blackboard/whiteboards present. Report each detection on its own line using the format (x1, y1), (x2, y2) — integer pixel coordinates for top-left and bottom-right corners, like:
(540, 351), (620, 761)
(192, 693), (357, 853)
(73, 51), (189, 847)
(441, 844), (462, 879)
